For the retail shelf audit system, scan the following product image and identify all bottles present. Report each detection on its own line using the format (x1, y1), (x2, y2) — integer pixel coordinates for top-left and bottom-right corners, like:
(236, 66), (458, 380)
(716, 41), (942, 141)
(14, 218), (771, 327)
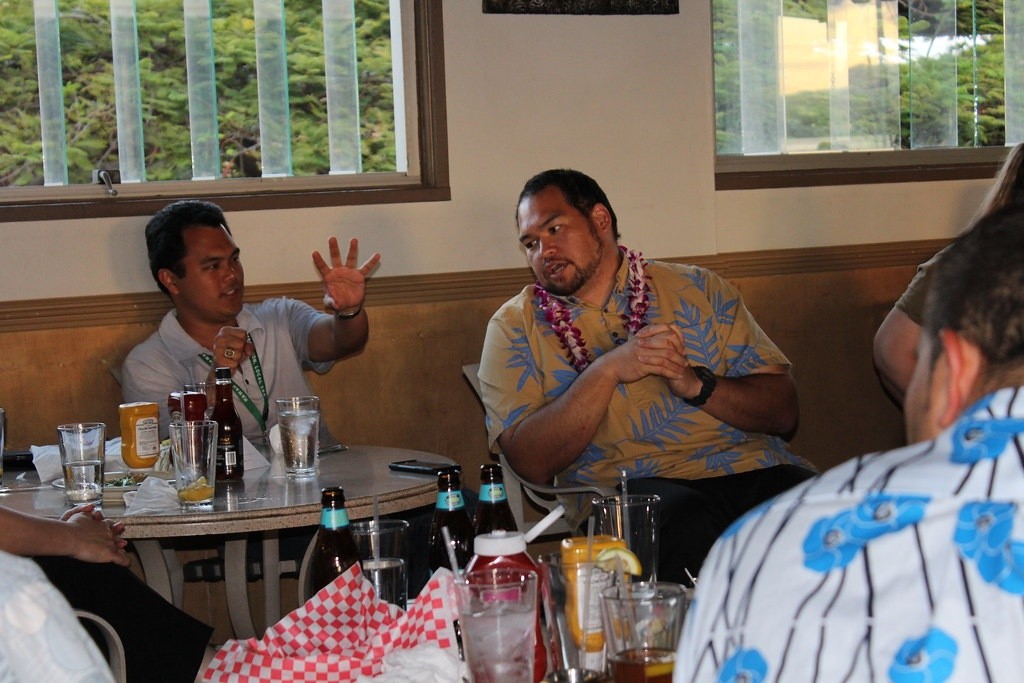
(425, 467), (473, 578)
(204, 366), (245, 485)
(473, 463), (520, 537)
(310, 486), (359, 598)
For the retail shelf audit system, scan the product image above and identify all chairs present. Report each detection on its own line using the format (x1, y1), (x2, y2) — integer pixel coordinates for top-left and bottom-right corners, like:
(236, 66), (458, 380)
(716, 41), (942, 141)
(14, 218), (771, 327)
(462, 362), (623, 543)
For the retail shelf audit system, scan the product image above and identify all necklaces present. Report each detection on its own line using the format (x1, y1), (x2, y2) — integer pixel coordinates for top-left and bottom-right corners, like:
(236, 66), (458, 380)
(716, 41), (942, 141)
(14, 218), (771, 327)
(534, 245), (652, 373)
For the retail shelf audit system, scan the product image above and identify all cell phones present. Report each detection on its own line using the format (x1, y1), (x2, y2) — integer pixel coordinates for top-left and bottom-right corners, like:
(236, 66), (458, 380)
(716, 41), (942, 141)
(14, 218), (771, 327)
(389, 459), (461, 477)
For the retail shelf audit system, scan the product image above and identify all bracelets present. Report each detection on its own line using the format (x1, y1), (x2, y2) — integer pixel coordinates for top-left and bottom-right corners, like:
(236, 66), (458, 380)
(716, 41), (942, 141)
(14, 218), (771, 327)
(335, 304), (363, 320)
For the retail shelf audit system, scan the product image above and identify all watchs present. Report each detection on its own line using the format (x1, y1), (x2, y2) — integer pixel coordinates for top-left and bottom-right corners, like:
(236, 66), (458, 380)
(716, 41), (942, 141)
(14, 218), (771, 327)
(682, 365), (718, 406)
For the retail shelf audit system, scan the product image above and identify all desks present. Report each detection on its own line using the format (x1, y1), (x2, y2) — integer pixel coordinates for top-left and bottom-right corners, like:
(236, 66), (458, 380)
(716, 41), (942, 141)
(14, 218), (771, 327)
(0, 444), (459, 641)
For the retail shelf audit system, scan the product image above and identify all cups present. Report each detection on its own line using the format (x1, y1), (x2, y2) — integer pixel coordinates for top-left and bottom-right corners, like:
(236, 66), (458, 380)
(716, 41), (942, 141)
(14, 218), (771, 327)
(591, 493), (662, 584)
(350, 518), (409, 610)
(452, 569), (539, 683)
(168, 419), (219, 512)
(275, 395), (320, 477)
(536, 548), (689, 683)
(56, 422), (106, 512)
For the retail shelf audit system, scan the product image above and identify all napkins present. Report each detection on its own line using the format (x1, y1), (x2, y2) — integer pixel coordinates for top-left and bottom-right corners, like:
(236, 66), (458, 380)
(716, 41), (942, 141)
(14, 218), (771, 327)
(29, 438), (132, 483)
(269, 423), (284, 453)
(356, 640), (470, 683)
(122, 477), (182, 515)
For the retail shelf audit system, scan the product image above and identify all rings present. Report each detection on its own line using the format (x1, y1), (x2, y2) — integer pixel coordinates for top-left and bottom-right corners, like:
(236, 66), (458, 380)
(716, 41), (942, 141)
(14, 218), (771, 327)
(224, 346), (234, 359)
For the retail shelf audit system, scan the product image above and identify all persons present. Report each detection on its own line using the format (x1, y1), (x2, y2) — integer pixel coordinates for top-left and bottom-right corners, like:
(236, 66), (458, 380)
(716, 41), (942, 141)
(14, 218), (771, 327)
(478, 169), (820, 589)
(670, 242), (1023, 683)
(873, 145), (1023, 384)
(1, 504), (218, 682)
(120, 198), (380, 448)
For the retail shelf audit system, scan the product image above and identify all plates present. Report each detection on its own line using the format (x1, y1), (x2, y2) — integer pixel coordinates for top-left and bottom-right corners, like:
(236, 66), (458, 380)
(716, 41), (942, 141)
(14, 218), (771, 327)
(51, 469), (177, 507)
